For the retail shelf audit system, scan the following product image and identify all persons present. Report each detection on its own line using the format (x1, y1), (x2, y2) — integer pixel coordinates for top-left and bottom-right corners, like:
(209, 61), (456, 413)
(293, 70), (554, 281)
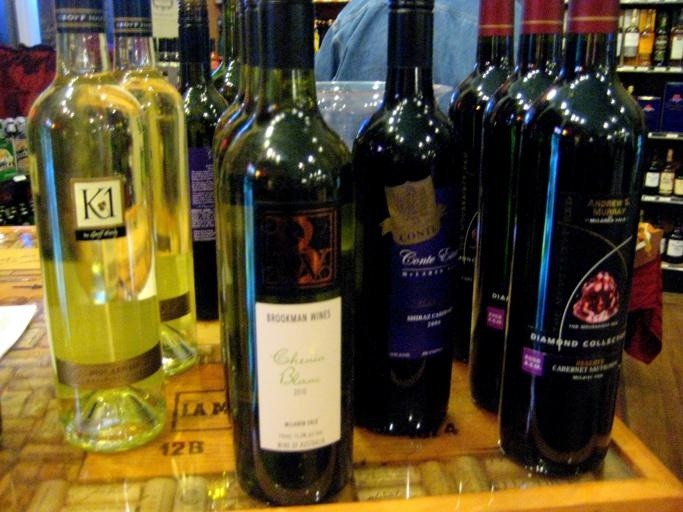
(315, 0), (482, 91)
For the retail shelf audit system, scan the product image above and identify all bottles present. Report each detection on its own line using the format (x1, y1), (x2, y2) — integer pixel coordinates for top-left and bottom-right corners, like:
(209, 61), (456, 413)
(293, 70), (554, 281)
(447, 1), (649, 476)
(615, 8), (683, 68)
(642, 149), (683, 198)
(23, 3), (201, 454)
(654, 219), (683, 265)
(352, 3), (463, 435)
(174, 0), (360, 505)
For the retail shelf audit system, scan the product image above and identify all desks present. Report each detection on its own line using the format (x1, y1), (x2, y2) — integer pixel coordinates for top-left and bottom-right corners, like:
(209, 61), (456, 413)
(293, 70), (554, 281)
(1, 224), (681, 512)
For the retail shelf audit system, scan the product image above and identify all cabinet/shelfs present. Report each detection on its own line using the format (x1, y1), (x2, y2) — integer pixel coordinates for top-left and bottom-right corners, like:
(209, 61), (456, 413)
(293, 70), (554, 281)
(611, 0), (683, 293)
